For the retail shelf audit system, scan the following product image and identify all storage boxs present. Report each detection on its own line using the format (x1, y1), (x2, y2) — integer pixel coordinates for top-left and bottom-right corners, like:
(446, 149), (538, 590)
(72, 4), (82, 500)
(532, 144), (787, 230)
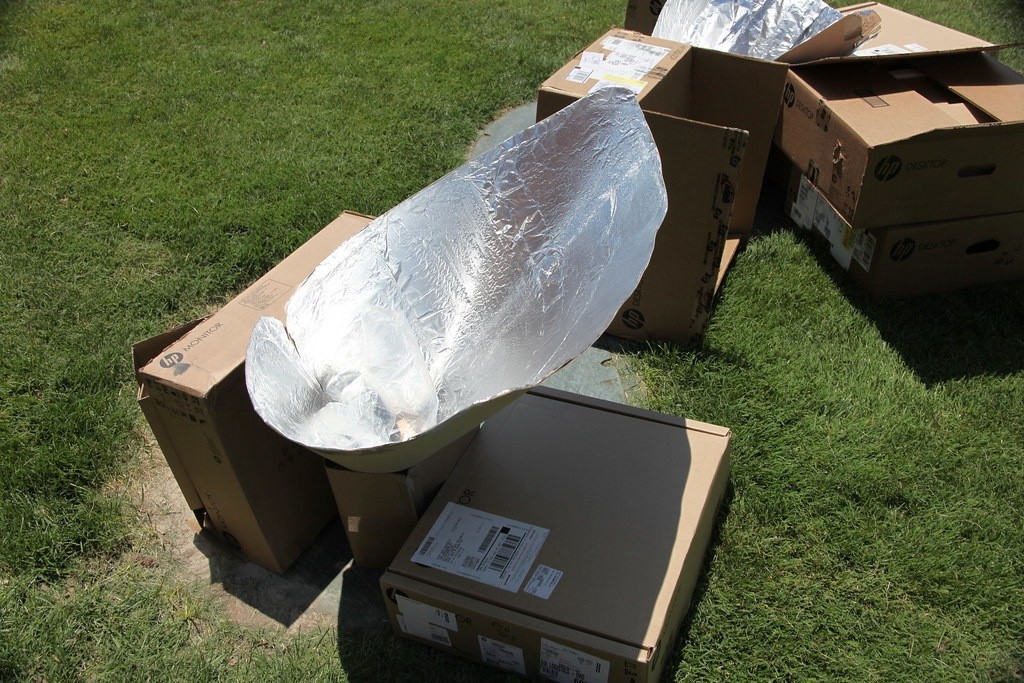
(317, 422), (482, 593)
(624, 0), (667, 37)
(767, 147), (1024, 301)
(835, 0), (998, 58)
(778, 42), (1024, 230)
(535, 27), (792, 348)
(130, 211), (378, 575)
(380, 386), (731, 683)
